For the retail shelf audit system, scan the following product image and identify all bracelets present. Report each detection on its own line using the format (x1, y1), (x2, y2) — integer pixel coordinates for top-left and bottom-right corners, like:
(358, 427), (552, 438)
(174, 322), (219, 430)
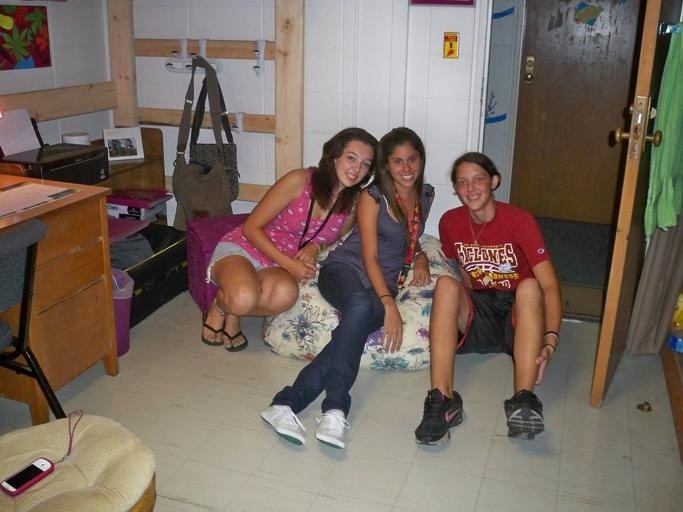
(542, 344), (555, 353)
(543, 331), (559, 343)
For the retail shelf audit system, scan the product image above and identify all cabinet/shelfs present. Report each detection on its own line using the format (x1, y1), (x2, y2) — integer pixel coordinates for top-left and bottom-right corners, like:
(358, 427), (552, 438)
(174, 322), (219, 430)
(0, 122), (168, 259)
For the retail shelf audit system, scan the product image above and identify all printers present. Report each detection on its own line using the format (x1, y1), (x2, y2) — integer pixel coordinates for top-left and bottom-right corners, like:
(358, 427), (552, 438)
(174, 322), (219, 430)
(0, 118), (110, 185)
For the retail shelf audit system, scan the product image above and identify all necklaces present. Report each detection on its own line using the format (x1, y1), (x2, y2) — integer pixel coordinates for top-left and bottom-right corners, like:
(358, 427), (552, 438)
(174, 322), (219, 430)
(465, 201), (493, 245)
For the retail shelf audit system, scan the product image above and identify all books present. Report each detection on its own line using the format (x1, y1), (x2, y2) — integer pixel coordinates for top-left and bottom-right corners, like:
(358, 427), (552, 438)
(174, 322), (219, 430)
(103, 188), (175, 245)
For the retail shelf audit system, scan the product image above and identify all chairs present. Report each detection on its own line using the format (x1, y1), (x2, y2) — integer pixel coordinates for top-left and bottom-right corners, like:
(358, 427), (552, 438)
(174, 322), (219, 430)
(0, 216), (72, 424)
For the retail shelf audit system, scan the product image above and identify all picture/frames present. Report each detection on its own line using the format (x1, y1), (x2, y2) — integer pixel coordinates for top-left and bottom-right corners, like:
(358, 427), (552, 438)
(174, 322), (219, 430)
(102, 126), (145, 162)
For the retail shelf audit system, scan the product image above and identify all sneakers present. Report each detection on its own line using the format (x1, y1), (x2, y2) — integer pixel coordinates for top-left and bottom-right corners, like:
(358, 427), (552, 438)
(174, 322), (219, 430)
(260, 405), (306, 446)
(415, 387), (464, 445)
(504, 389), (544, 440)
(314, 409), (350, 449)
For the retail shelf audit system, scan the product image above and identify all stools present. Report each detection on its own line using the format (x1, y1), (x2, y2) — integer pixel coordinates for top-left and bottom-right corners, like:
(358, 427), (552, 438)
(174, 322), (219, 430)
(179, 210), (257, 322)
(0, 407), (157, 511)
(254, 226), (464, 373)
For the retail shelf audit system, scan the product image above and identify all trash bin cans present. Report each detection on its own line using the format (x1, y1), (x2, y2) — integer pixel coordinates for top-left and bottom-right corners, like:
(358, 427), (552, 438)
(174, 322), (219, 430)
(111, 267), (135, 356)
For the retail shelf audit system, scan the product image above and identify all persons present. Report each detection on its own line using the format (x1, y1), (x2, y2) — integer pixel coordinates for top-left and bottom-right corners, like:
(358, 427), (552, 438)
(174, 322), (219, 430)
(379, 295), (394, 299)
(323, 244), (325, 251)
(201, 127), (380, 352)
(259, 127), (436, 449)
(313, 242), (321, 254)
(414, 250), (424, 260)
(415, 152), (564, 441)
(110, 139), (137, 158)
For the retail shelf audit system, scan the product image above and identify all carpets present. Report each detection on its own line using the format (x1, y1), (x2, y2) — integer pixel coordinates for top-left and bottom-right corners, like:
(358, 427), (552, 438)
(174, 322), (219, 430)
(521, 206), (617, 297)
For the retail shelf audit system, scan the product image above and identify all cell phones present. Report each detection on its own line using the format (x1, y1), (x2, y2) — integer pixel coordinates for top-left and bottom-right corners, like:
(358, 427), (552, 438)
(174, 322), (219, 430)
(0, 457), (54, 497)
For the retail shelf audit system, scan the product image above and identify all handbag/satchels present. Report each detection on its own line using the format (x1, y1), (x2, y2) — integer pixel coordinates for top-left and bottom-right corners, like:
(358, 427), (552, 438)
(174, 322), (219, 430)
(190, 143), (239, 202)
(172, 154), (233, 232)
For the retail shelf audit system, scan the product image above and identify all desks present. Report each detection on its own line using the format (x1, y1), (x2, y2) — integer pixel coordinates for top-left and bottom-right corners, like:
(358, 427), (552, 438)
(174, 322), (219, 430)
(0, 171), (120, 432)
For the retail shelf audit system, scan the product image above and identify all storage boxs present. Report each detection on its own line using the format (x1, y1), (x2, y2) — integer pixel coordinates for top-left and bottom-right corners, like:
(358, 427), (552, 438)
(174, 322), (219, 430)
(95, 217), (192, 331)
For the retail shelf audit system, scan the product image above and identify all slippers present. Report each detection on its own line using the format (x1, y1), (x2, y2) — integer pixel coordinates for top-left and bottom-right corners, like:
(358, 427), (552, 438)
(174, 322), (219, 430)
(223, 330), (248, 352)
(202, 310), (224, 346)
